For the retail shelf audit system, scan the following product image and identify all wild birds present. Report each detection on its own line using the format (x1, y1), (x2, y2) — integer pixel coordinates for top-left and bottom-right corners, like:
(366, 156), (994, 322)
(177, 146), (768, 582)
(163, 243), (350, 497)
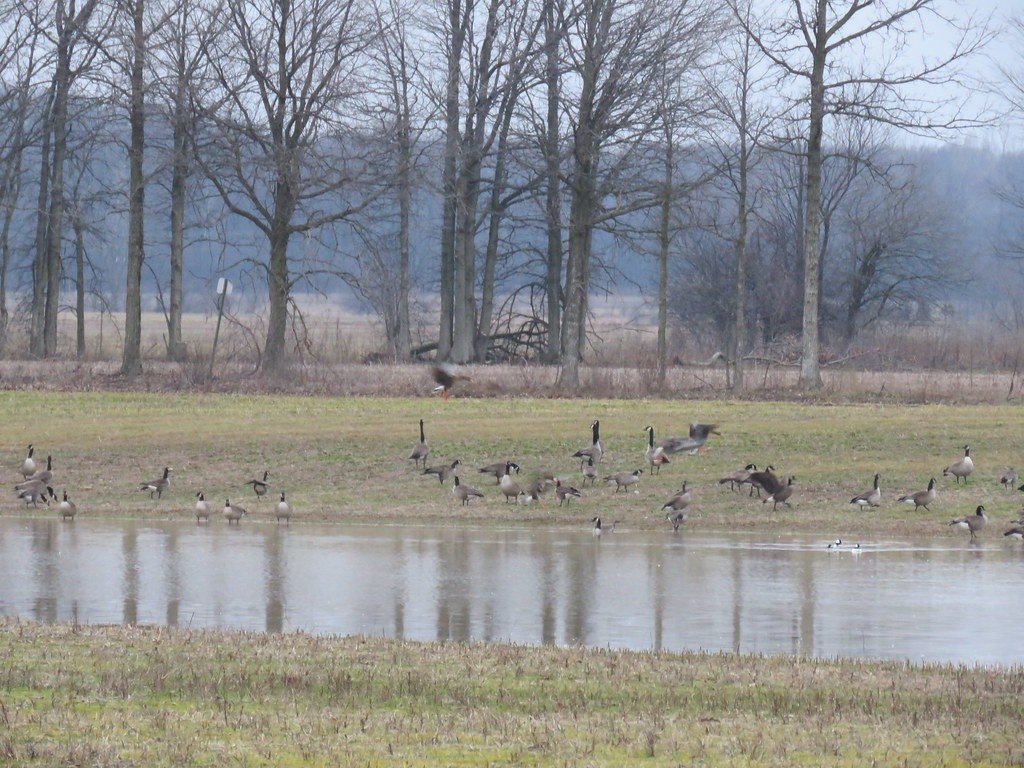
(194, 491), (211, 521)
(849, 472), (883, 512)
(223, 499), (247, 526)
(897, 477), (939, 512)
(1003, 502), (1024, 541)
(243, 470), (272, 503)
(406, 418), (798, 536)
(999, 466), (1024, 492)
(276, 490), (292, 528)
(826, 539), (862, 552)
(14, 443), (77, 521)
(430, 365), (472, 402)
(943, 444), (975, 484)
(947, 505), (989, 539)
(138, 466), (174, 499)
